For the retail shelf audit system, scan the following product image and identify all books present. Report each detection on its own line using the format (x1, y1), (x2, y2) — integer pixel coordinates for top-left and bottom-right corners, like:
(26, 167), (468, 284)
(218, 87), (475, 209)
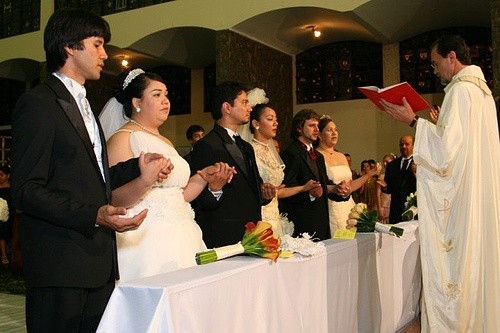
(357, 82), (432, 113)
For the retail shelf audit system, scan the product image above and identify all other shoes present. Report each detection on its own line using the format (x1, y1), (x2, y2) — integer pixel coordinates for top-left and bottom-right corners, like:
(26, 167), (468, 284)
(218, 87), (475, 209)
(1, 257), (9, 264)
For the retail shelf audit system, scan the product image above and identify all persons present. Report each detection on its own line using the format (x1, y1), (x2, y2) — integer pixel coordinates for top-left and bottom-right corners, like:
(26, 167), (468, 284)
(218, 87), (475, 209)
(345, 153), (397, 225)
(378, 34), (500, 333)
(315, 114), (383, 237)
(280, 108), (351, 242)
(237, 87), (320, 238)
(98, 68), (237, 280)
(191, 79), (277, 250)
(186, 125), (205, 147)
(11, 7), (174, 333)
(376, 133), (416, 225)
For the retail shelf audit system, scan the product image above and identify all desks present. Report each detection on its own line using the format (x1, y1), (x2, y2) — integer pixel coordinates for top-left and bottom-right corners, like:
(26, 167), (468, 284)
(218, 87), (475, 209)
(95, 220), (425, 332)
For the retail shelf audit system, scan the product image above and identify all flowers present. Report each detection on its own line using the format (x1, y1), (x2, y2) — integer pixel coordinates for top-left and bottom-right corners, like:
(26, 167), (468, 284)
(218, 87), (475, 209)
(402, 193), (419, 220)
(347, 202), (404, 237)
(195, 220), (292, 264)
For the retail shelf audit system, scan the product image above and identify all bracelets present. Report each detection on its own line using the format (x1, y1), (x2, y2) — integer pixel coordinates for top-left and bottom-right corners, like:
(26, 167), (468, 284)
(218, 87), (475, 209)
(409, 115), (419, 127)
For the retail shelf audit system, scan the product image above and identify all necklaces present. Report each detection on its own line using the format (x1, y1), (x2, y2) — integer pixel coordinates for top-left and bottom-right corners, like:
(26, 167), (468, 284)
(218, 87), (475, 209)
(252, 138), (268, 147)
(130, 120), (159, 135)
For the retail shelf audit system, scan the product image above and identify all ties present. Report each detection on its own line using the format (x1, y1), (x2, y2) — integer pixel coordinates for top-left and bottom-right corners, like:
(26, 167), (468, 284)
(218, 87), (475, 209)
(309, 146), (317, 163)
(234, 135), (251, 174)
(402, 159), (409, 170)
(78, 89), (106, 184)
